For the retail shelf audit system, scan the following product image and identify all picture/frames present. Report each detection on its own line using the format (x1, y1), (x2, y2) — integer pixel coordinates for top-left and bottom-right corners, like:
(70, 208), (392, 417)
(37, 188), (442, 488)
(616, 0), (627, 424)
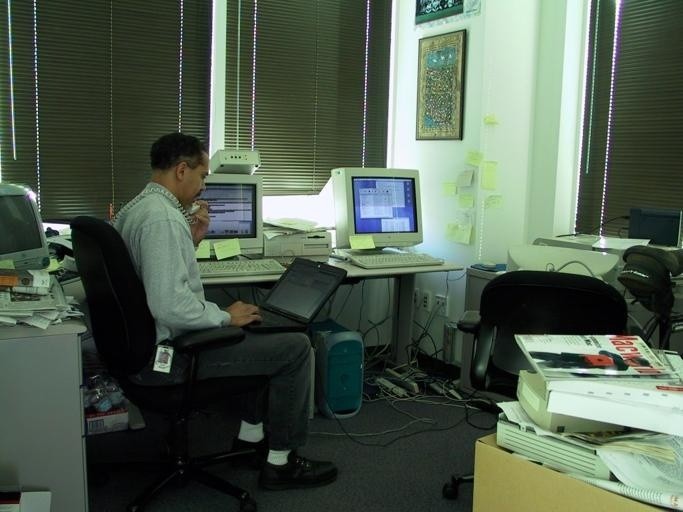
(415, 28), (467, 142)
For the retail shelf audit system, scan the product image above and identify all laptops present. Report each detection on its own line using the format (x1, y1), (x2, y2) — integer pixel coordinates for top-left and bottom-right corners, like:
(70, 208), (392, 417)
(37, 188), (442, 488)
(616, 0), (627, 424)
(244, 257), (347, 333)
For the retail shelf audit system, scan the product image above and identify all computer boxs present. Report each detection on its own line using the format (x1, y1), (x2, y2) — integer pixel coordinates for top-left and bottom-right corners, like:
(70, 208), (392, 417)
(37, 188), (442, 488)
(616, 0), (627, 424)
(307, 317), (364, 419)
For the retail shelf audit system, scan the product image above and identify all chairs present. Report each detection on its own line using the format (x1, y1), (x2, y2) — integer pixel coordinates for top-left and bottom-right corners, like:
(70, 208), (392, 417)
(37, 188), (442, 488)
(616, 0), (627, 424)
(443, 271), (628, 501)
(70, 216), (270, 511)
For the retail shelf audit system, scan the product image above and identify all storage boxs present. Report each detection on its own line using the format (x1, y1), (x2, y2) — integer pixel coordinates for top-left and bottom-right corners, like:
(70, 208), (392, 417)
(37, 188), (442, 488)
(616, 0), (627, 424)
(471, 432), (673, 512)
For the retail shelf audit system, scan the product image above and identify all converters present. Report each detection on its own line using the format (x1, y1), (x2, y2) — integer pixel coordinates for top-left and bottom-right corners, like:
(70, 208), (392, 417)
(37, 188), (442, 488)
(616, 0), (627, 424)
(429, 383), (447, 395)
(364, 378), (378, 397)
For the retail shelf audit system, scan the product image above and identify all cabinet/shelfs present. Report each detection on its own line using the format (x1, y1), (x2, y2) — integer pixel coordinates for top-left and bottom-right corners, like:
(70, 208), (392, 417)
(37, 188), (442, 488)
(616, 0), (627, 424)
(1, 314), (87, 512)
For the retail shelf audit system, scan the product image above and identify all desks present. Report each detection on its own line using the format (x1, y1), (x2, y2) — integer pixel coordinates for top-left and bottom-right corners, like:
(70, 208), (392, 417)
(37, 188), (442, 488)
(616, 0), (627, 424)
(203, 248), (464, 377)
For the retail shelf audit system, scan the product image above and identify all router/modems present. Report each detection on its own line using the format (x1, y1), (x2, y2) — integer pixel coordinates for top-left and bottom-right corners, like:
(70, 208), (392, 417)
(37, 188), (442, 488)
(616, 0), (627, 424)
(209, 150), (261, 173)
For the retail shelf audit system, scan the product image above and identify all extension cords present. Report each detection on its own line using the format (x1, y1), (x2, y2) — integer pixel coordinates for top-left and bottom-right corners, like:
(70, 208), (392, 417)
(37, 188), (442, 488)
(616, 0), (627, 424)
(377, 377), (406, 398)
(385, 368), (419, 393)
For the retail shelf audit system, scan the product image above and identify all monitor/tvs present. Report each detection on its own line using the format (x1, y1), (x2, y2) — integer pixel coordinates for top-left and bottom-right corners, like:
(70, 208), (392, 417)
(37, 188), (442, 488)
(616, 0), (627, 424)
(0, 183), (50, 276)
(318, 167), (423, 257)
(194, 174), (264, 260)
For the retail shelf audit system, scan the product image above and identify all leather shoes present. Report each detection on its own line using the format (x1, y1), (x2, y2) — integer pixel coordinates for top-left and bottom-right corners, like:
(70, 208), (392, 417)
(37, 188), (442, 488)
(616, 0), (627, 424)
(257, 448), (338, 490)
(232, 435), (301, 470)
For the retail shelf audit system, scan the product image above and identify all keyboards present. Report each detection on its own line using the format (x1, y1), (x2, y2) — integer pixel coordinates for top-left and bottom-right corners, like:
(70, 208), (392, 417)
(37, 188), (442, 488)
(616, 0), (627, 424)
(351, 252), (444, 269)
(198, 259), (286, 285)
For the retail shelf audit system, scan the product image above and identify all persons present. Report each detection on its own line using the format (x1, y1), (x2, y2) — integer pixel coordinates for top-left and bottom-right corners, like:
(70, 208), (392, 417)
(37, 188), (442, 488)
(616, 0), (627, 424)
(107, 131), (337, 491)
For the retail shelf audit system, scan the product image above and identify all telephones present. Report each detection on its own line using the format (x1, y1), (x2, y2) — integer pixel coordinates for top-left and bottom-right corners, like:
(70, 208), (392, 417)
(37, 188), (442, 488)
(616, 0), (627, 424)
(185, 200), (200, 215)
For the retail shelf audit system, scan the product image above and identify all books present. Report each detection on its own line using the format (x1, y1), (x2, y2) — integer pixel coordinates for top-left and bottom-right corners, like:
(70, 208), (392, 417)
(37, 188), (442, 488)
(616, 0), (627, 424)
(1, 269), (51, 288)
(497, 334), (683, 512)
